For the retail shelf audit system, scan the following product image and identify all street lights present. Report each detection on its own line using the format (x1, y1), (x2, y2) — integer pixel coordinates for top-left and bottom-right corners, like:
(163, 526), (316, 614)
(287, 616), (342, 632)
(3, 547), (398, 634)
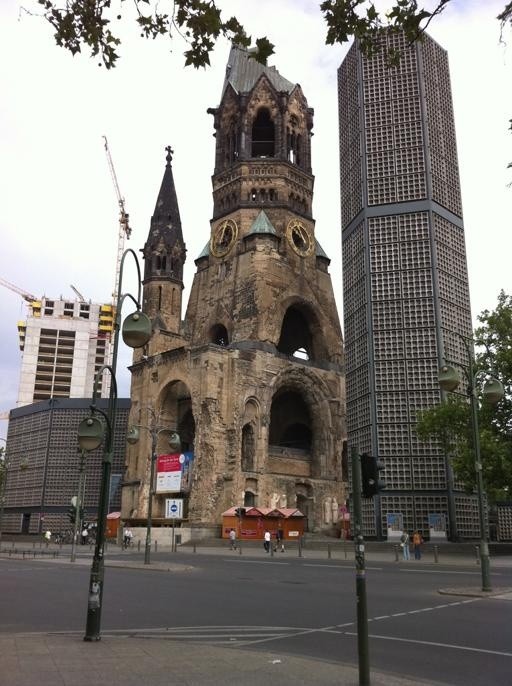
(128, 408), (181, 565)
(437, 330), (504, 592)
(0, 436), (29, 531)
(77, 248), (154, 640)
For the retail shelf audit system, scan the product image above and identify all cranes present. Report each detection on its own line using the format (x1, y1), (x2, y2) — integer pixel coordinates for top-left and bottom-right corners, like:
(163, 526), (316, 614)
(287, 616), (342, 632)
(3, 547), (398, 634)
(0, 277), (39, 305)
(97, 132), (132, 397)
(69, 285), (92, 303)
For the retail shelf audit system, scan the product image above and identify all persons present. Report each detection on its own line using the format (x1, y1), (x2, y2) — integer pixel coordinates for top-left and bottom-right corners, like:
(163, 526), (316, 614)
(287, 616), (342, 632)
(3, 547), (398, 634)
(273, 523), (285, 552)
(325, 498), (331, 523)
(332, 498), (338, 524)
(264, 529), (271, 553)
(82, 529), (88, 545)
(125, 528), (132, 549)
(281, 494), (287, 508)
(400, 531), (410, 560)
(230, 528), (236, 550)
(413, 532), (421, 559)
(270, 494), (280, 509)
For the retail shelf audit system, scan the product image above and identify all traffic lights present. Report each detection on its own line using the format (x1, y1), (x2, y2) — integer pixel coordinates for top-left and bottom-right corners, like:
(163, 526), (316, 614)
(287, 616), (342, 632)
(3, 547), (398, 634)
(362, 454), (387, 498)
